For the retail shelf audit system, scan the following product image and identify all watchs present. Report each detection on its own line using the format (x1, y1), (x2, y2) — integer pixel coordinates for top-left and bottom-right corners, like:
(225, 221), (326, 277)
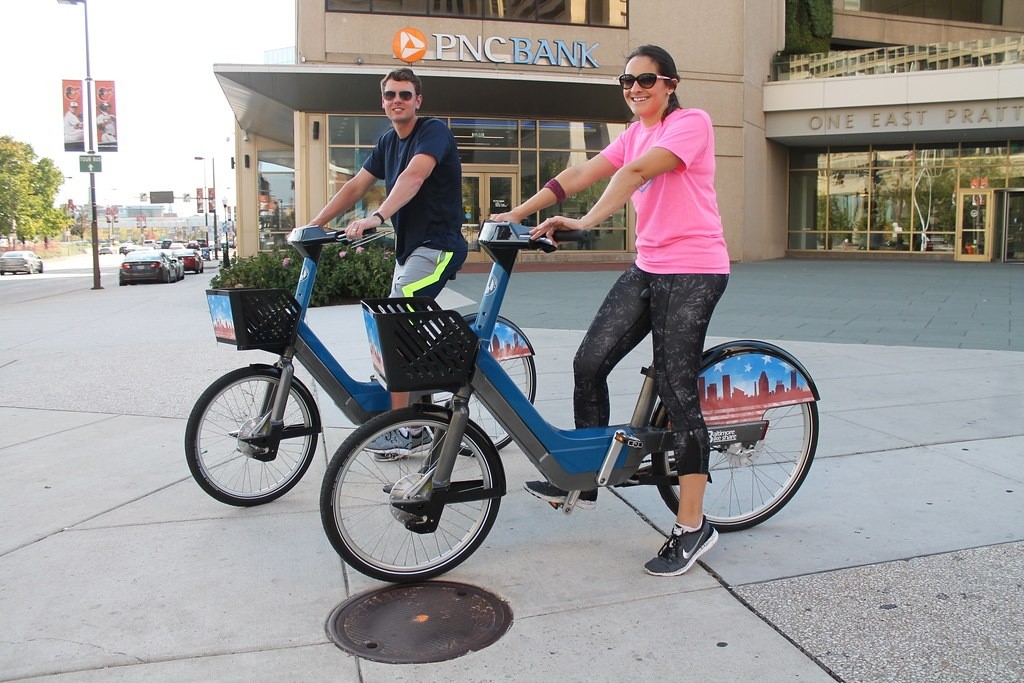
(373, 212), (384, 225)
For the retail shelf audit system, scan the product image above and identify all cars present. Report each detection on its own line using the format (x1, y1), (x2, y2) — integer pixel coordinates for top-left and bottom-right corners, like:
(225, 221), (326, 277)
(118, 251), (177, 286)
(119, 243), (135, 254)
(0, 251), (44, 275)
(99, 242), (117, 255)
(143, 240), (237, 261)
(174, 250), (204, 275)
(154, 249), (185, 280)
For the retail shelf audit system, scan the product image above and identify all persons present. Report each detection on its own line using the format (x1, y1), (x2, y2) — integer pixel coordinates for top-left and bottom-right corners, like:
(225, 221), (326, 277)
(97, 102), (116, 142)
(306, 68), (468, 463)
(197, 191), (202, 210)
(488, 41), (731, 576)
(64, 102), (84, 141)
(209, 191), (215, 210)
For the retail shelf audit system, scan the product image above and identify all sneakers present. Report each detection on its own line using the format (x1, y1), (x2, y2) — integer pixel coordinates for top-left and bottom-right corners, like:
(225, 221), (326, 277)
(362, 427), (433, 462)
(522, 480), (601, 510)
(643, 516), (721, 576)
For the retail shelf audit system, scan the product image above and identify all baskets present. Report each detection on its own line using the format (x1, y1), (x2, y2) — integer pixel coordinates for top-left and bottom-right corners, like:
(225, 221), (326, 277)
(206, 287), (303, 350)
(363, 295), (480, 392)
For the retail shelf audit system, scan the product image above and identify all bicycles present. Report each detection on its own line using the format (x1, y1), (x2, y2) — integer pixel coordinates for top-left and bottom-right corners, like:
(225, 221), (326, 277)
(183, 226), (538, 509)
(318, 220), (822, 586)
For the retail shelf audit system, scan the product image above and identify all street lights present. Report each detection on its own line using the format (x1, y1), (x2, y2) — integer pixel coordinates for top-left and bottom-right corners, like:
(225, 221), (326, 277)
(195, 157), (218, 260)
(57, 0), (103, 290)
(222, 195), (228, 253)
(44, 176), (73, 249)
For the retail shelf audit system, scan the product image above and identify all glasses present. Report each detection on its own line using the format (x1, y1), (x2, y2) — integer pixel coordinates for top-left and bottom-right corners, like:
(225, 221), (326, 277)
(381, 91), (418, 101)
(617, 72), (673, 90)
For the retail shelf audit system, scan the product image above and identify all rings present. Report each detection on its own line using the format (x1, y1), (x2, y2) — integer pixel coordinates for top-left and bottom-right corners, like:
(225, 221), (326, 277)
(352, 229), (357, 232)
